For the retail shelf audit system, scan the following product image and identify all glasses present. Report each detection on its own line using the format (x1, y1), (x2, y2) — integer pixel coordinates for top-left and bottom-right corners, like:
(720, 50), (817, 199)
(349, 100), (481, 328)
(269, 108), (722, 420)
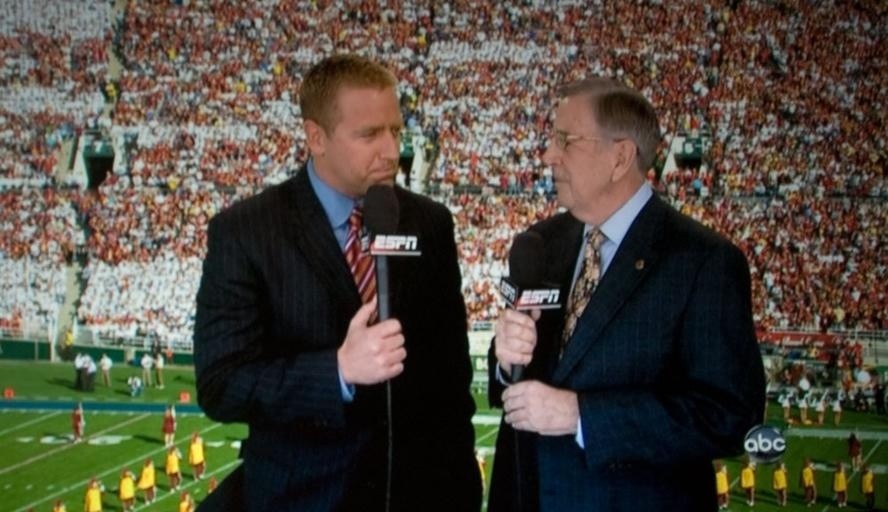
(551, 130), (598, 148)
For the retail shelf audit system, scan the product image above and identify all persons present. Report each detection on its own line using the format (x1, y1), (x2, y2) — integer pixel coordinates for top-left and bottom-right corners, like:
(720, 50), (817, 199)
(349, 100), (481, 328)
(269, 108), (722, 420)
(0, 0), (888, 512)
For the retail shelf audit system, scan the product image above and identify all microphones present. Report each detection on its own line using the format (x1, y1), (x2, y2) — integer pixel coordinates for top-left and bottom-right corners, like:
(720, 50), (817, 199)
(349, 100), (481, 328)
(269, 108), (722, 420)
(359, 184), (421, 322)
(500, 232), (562, 384)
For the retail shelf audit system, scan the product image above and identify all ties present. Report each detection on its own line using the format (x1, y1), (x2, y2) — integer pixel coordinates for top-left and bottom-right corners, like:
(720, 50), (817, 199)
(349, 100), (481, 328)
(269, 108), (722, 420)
(344, 207), (379, 323)
(559, 232), (606, 362)
